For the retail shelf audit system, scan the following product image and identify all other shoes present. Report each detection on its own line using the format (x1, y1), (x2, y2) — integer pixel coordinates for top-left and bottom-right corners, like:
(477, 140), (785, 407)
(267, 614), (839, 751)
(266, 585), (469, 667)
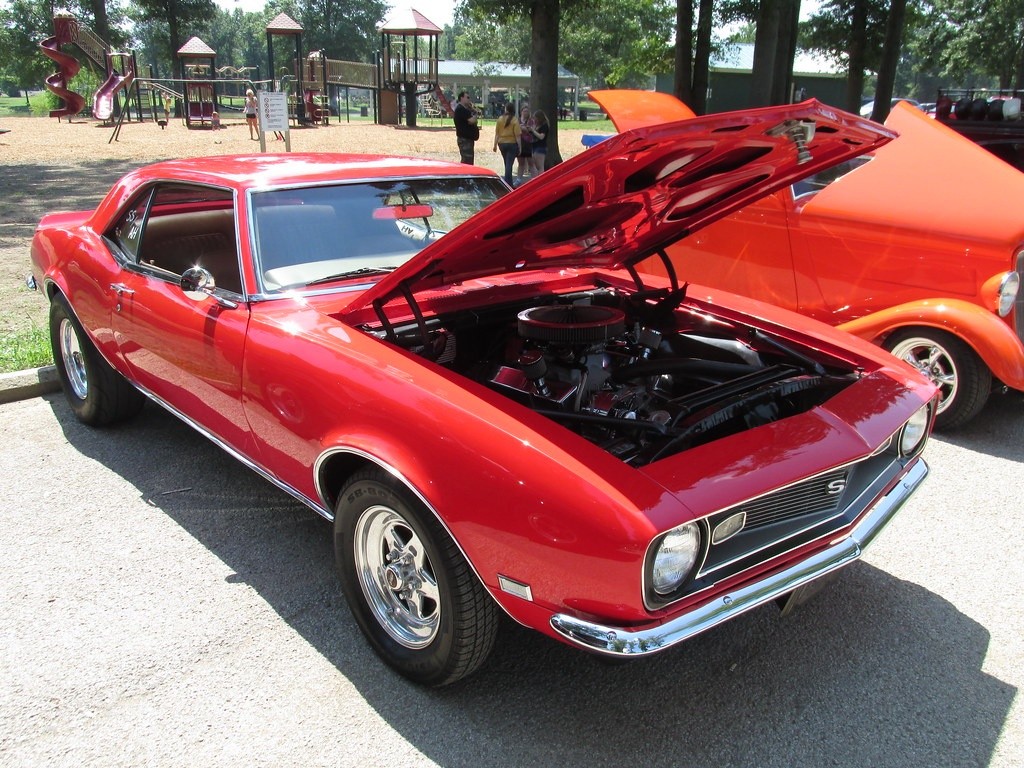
(513, 179), (524, 187)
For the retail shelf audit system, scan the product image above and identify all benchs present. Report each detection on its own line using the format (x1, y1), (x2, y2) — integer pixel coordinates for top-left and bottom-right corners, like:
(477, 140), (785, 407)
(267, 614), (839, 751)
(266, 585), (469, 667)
(133, 204), (337, 291)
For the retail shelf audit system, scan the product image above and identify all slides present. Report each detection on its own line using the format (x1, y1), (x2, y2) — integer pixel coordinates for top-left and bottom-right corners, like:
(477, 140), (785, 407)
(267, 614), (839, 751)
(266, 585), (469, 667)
(38, 35), (86, 118)
(301, 89), (324, 126)
(433, 83), (456, 119)
(92, 70), (132, 121)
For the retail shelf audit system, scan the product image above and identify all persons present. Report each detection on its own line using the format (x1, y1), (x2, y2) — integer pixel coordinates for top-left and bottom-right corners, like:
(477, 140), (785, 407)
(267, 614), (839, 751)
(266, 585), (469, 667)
(492, 102), (523, 188)
(511, 106), (534, 186)
(453, 92), (479, 166)
(242, 89), (260, 141)
(492, 100), (502, 119)
(162, 93), (171, 122)
(211, 112), (220, 131)
(527, 110), (550, 175)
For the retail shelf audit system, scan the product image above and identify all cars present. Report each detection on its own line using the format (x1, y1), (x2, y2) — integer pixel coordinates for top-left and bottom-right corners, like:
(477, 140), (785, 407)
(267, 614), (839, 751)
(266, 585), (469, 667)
(586, 88), (1024, 432)
(30, 99), (941, 689)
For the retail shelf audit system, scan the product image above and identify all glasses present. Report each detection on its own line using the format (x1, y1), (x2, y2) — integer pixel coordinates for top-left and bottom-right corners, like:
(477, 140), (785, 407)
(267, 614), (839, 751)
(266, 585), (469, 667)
(464, 96), (470, 99)
